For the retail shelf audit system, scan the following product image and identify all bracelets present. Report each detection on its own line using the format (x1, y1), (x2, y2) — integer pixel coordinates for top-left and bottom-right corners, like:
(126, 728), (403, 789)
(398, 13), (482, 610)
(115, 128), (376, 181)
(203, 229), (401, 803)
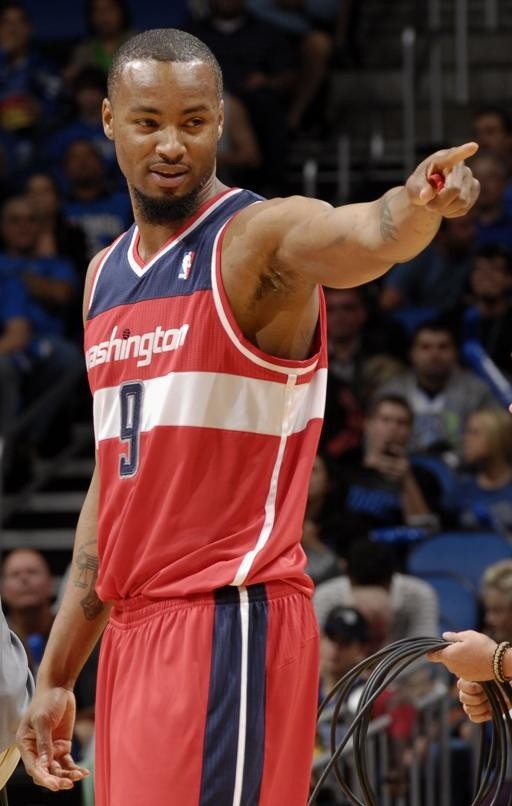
(492, 640), (509, 683)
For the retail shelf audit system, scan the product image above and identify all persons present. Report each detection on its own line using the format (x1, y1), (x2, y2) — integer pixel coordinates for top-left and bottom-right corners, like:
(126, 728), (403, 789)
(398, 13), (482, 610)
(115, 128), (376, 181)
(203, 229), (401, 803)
(1, 546), (94, 805)
(319, 604), (474, 805)
(16, 31), (480, 806)
(1, 0), (512, 641)
(426, 629), (512, 723)
